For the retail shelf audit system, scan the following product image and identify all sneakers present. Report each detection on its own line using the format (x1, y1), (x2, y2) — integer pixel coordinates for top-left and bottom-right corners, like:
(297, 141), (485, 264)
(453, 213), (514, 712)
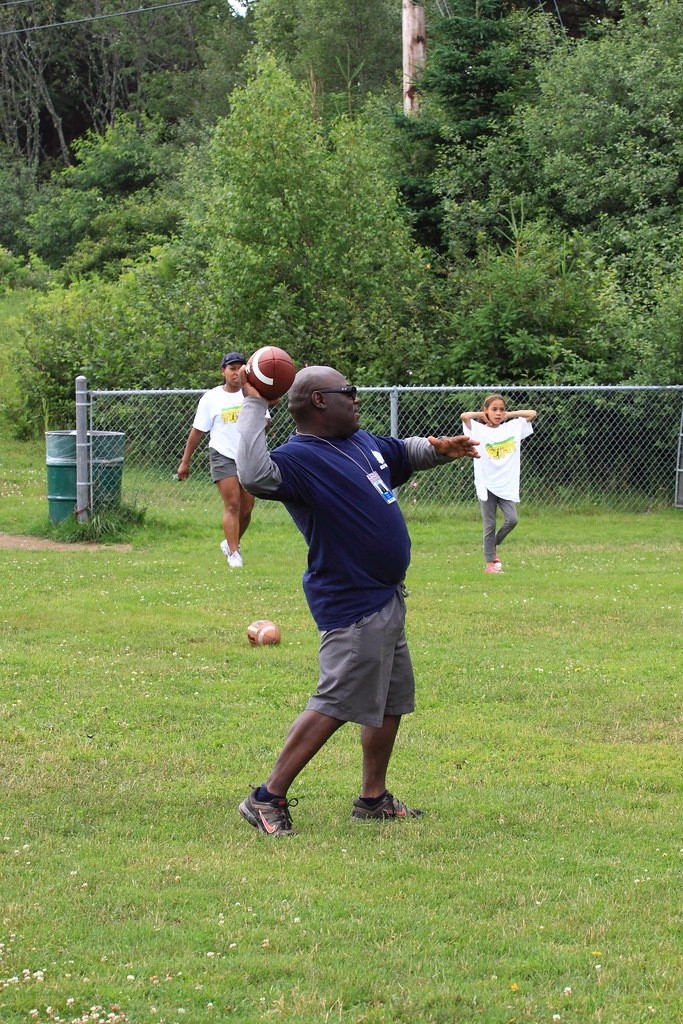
(240, 786), (296, 837)
(485, 561), (504, 574)
(348, 790), (425, 822)
(221, 539), (243, 568)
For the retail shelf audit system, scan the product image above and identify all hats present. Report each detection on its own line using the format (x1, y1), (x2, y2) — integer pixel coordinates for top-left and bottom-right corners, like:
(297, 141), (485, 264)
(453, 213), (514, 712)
(222, 351), (246, 365)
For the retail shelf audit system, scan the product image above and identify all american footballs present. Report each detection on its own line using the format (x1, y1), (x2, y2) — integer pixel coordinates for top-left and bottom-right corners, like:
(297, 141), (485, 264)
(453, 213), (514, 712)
(244, 345), (295, 398)
(247, 620), (281, 646)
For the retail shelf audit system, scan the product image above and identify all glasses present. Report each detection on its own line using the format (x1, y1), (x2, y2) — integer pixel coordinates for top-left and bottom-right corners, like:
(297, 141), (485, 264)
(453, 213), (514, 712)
(319, 386), (357, 399)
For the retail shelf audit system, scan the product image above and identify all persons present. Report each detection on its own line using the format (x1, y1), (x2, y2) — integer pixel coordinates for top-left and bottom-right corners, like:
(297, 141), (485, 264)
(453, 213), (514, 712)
(176, 352), (271, 569)
(235, 365), (481, 837)
(459, 394), (536, 575)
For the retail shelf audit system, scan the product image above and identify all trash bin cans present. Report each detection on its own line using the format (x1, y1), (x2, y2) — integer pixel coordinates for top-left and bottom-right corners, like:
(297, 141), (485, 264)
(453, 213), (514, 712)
(44, 429), (126, 526)
